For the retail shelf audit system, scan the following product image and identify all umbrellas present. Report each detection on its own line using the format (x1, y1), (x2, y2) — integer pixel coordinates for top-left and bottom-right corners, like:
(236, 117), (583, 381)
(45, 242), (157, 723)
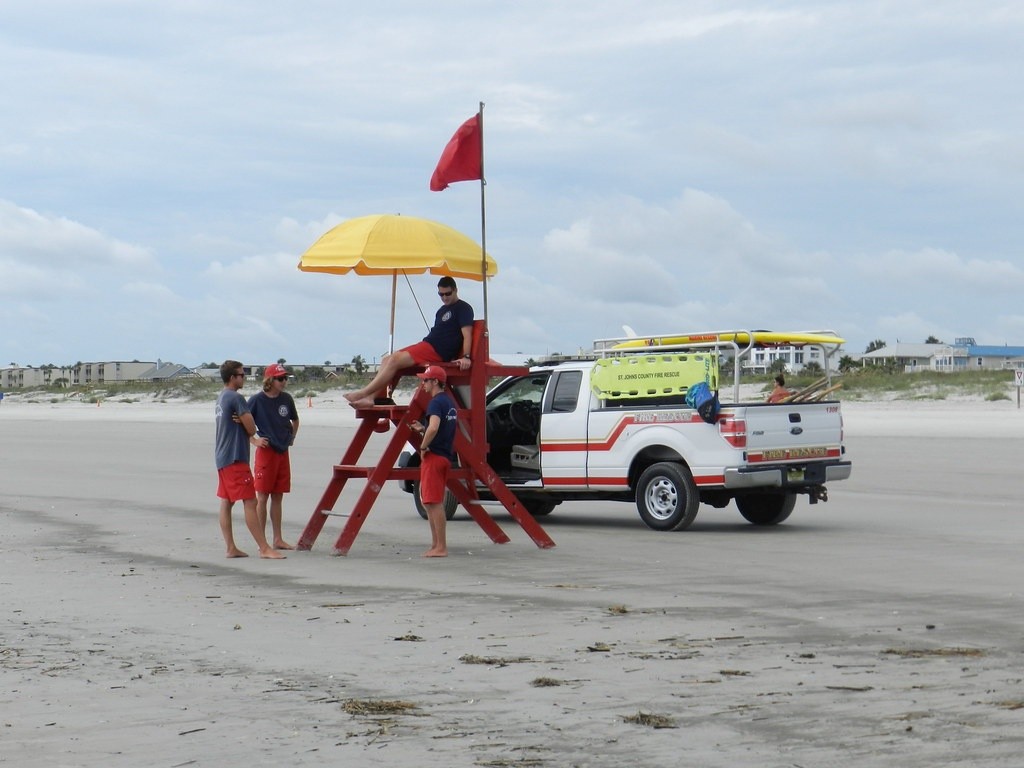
(298, 214), (497, 399)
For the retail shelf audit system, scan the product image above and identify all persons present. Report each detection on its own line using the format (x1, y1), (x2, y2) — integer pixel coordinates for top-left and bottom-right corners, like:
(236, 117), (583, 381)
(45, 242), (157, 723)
(767, 374), (788, 402)
(342, 276), (474, 410)
(406, 366), (457, 557)
(215, 360), (286, 558)
(247, 364), (299, 550)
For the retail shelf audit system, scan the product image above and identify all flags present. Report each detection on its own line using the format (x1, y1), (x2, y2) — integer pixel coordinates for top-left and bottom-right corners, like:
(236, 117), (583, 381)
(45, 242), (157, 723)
(430, 112), (480, 192)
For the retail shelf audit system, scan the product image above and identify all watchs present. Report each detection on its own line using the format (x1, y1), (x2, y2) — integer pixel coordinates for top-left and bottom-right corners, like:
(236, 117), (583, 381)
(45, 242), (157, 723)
(463, 354), (470, 359)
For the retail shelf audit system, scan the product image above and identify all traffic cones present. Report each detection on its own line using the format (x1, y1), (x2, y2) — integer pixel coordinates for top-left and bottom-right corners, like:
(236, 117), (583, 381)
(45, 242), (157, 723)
(97, 399), (100, 406)
(307, 396), (313, 407)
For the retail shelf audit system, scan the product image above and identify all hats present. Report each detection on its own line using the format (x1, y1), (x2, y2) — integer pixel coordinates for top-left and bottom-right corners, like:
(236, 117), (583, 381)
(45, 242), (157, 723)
(265, 363), (289, 377)
(416, 366), (446, 383)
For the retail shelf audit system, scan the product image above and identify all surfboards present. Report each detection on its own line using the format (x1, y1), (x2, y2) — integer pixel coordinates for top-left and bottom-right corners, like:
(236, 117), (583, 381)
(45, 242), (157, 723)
(611, 324), (847, 348)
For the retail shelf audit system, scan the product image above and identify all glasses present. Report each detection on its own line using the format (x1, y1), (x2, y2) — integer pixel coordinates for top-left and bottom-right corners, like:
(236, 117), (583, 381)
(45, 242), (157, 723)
(232, 373), (244, 378)
(274, 376), (288, 381)
(423, 378), (434, 382)
(438, 289), (454, 296)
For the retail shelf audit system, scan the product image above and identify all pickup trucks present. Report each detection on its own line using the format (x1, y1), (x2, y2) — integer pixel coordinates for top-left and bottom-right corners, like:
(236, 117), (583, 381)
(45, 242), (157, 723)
(398, 328), (853, 530)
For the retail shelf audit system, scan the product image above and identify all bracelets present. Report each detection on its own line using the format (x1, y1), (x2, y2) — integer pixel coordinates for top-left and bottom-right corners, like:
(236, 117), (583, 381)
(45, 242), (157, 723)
(420, 448), (426, 450)
